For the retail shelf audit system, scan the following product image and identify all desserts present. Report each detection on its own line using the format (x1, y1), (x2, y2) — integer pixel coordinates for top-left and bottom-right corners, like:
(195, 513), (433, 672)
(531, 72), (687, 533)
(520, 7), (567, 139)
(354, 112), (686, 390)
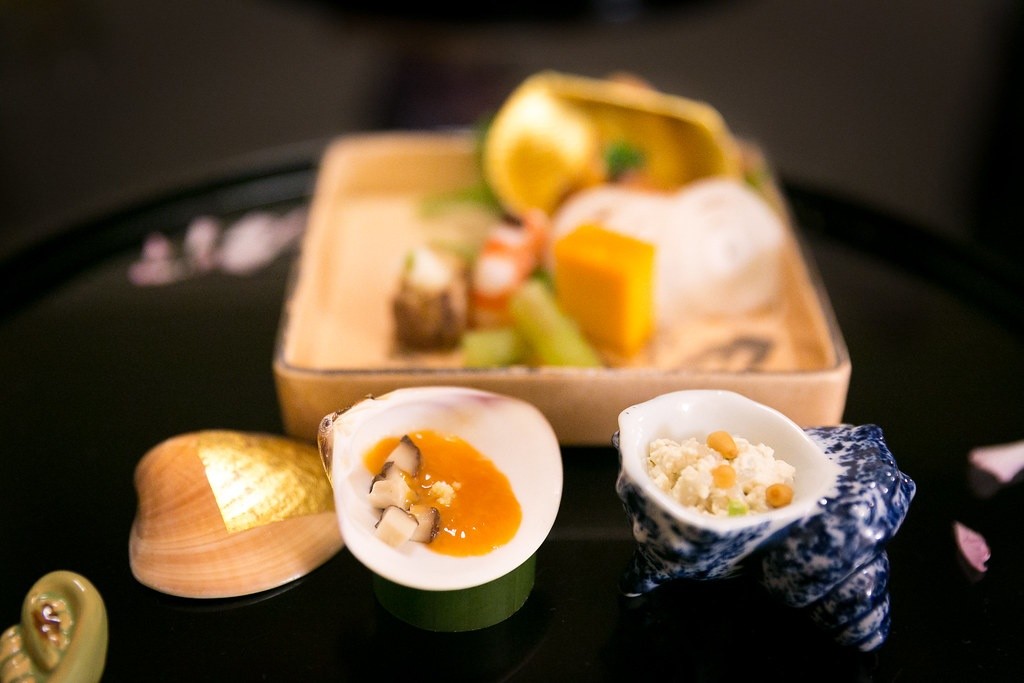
(642, 429), (798, 518)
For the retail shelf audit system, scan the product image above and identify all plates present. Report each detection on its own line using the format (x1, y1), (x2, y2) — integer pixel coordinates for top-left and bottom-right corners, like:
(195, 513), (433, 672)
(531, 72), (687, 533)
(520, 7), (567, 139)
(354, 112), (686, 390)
(273, 127), (852, 448)
(0, 146), (1024, 683)
